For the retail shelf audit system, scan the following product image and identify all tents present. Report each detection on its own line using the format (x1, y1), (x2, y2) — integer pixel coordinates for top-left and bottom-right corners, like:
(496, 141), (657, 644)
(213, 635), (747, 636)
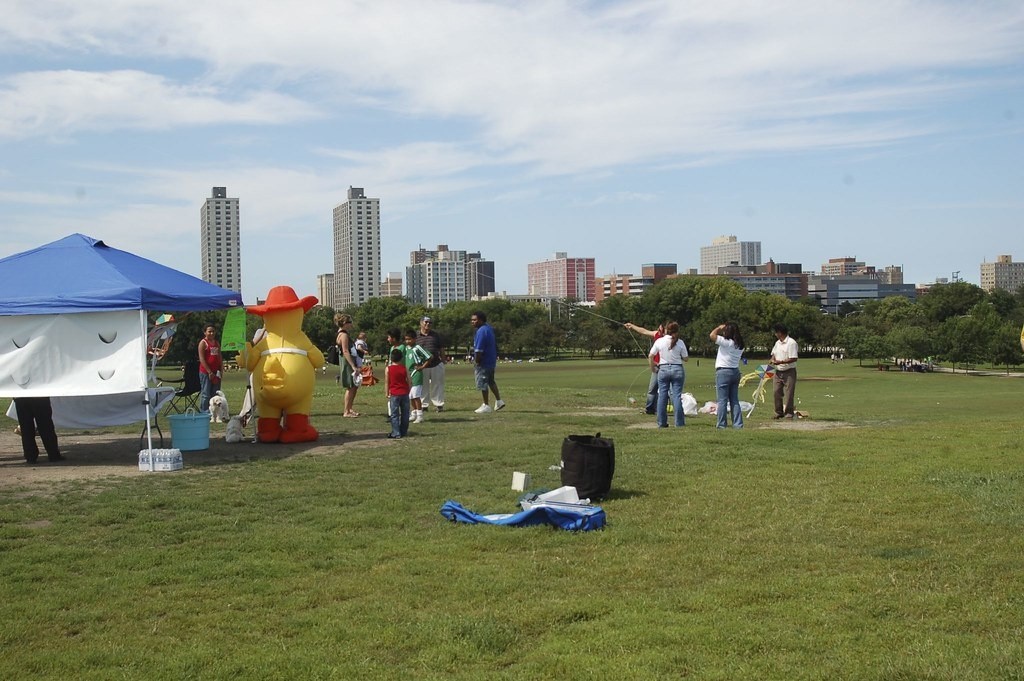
(0, 233), (257, 472)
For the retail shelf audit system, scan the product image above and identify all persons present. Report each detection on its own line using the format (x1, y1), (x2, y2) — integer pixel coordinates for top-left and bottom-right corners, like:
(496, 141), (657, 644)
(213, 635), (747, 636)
(386, 329), (433, 423)
(625, 321), (668, 414)
(198, 324), (224, 412)
(253, 324), (267, 344)
(472, 311), (506, 413)
(648, 321), (689, 428)
(463, 355), (474, 364)
(334, 313), (361, 418)
(899, 360), (911, 370)
(416, 316), (445, 413)
(770, 322), (799, 419)
(14, 397), (66, 464)
(355, 333), (368, 366)
(880, 365), (890, 371)
(710, 321), (745, 429)
(831, 354), (843, 363)
(447, 356), (454, 363)
(384, 349), (411, 439)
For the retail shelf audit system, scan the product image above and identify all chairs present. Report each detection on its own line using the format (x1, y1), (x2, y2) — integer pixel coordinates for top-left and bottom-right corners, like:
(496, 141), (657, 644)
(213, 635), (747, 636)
(165, 361), (201, 415)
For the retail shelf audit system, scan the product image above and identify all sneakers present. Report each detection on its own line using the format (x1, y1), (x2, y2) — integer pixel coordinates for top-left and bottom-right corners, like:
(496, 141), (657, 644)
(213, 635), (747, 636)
(494, 399), (506, 411)
(409, 416), (416, 422)
(474, 403), (492, 413)
(413, 418), (424, 424)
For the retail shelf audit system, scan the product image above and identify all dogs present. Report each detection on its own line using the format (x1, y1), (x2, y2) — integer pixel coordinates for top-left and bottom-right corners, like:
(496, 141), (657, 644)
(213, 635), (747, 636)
(225, 415), (243, 443)
(209, 390), (230, 423)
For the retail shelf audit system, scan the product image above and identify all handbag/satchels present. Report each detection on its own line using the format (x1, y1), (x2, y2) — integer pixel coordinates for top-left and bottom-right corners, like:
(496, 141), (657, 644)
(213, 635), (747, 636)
(327, 346), (339, 366)
(559, 432), (615, 504)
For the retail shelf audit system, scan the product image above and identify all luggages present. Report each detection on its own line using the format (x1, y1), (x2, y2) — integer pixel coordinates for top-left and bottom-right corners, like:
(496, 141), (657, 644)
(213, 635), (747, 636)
(359, 353), (374, 387)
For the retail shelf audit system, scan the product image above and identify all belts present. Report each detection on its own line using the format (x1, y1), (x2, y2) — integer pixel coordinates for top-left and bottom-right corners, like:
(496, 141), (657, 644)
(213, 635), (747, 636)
(717, 367), (737, 370)
(659, 363), (682, 366)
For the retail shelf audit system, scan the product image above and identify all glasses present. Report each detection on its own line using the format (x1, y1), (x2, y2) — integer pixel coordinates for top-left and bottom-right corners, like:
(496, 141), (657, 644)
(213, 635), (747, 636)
(346, 320), (353, 325)
(421, 320), (433, 325)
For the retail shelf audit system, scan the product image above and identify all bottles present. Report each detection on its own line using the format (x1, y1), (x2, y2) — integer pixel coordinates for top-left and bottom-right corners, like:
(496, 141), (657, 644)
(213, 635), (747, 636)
(139, 449), (183, 463)
(163, 464), (169, 469)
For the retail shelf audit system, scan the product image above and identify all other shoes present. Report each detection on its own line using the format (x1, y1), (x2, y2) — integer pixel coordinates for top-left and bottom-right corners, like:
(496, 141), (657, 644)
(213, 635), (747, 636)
(387, 431), (400, 439)
(437, 406), (443, 412)
(27, 455), (38, 464)
(774, 414), (784, 419)
(423, 407), (429, 411)
(48, 455), (66, 462)
(342, 410), (360, 417)
(786, 412), (793, 419)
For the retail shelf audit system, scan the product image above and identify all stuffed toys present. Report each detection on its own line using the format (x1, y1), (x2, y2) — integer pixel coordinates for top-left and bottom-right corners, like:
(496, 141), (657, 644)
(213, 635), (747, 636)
(237, 286), (326, 444)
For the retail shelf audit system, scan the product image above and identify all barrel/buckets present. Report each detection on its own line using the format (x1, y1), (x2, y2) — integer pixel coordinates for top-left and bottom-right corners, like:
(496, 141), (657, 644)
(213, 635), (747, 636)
(167, 408), (212, 450)
